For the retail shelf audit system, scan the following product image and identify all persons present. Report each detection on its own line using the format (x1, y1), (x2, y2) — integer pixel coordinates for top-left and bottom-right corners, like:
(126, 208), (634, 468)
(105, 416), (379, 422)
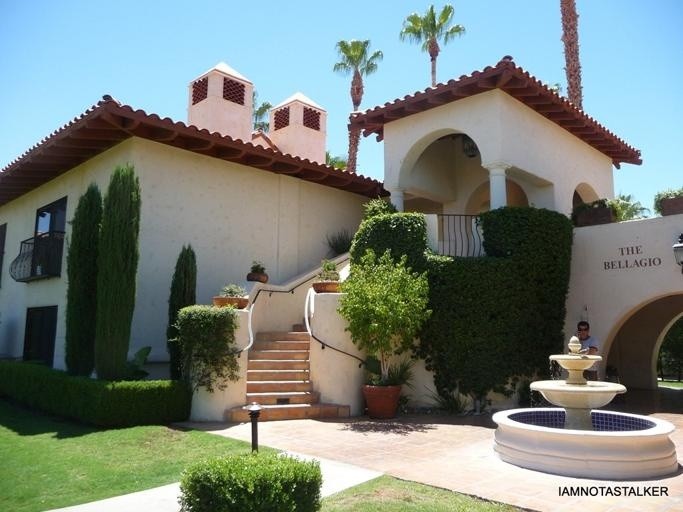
(567, 320), (599, 381)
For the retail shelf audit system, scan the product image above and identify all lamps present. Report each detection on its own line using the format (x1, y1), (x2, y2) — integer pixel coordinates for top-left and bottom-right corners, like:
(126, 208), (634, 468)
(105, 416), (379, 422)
(672, 232), (683, 274)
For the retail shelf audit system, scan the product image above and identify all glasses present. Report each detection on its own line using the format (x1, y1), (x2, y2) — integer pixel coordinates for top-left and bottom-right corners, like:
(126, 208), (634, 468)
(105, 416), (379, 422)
(579, 327), (589, 332)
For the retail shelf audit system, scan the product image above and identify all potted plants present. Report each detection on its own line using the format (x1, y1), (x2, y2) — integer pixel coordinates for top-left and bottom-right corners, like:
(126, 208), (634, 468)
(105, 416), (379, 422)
(572, 188), (682, 227)
(336, 247), (434, 418)
(212, 282), (249, 308)
(247, 259), (269, 284)
(312, 255), (344, 293)
